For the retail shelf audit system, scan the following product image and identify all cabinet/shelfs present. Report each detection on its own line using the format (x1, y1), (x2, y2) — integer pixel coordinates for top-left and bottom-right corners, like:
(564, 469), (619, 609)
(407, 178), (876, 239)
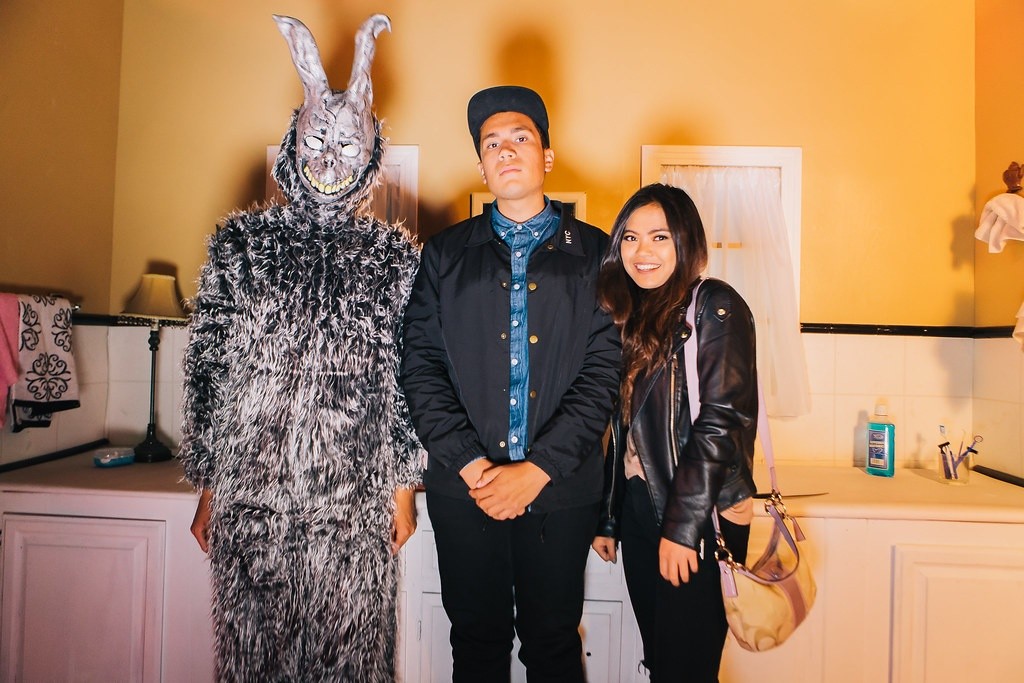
(0, 513), (167, 683)
(420, 531), (1023, 683)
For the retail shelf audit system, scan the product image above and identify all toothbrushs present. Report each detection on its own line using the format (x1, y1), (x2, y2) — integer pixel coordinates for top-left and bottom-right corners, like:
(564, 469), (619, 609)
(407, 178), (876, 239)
(938, 424), (947, 442)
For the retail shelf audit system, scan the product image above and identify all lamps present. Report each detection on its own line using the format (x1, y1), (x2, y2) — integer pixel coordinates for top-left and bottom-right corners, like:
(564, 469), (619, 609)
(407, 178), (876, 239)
(117, 273), (192, 462)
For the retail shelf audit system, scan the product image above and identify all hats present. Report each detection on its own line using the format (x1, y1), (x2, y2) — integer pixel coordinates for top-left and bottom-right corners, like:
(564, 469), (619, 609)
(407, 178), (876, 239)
(467, 86), (550, 163)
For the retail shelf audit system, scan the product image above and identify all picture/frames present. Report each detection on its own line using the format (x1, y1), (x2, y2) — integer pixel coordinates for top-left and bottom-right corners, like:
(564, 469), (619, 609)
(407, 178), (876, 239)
(471, 191), (587, 224)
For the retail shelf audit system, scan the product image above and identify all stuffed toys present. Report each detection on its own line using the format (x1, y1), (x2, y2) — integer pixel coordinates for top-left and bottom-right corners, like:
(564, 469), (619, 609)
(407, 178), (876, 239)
(179, 13), (427, 683)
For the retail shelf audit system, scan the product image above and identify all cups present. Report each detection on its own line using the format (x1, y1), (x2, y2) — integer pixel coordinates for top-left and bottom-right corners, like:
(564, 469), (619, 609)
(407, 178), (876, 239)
(938, 455), (969, 486)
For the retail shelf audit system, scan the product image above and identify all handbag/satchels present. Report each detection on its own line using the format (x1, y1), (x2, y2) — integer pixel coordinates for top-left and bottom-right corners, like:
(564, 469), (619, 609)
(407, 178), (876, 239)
(714, 492), (818, 654)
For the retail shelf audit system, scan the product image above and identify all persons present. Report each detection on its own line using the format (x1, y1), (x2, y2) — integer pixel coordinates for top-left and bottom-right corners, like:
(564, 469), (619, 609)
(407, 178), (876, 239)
(592, 183), (759, 683)
(402, 84), (628, 682)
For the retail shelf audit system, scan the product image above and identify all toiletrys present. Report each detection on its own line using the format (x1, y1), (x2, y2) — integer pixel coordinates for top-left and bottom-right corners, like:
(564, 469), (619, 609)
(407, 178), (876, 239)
(863, 404), (897, 477)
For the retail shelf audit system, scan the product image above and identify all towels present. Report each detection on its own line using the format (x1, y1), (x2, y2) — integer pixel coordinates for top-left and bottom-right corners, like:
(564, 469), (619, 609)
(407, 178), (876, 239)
(0, 292), (81, 433)
(974, 192), (1024, 353)
